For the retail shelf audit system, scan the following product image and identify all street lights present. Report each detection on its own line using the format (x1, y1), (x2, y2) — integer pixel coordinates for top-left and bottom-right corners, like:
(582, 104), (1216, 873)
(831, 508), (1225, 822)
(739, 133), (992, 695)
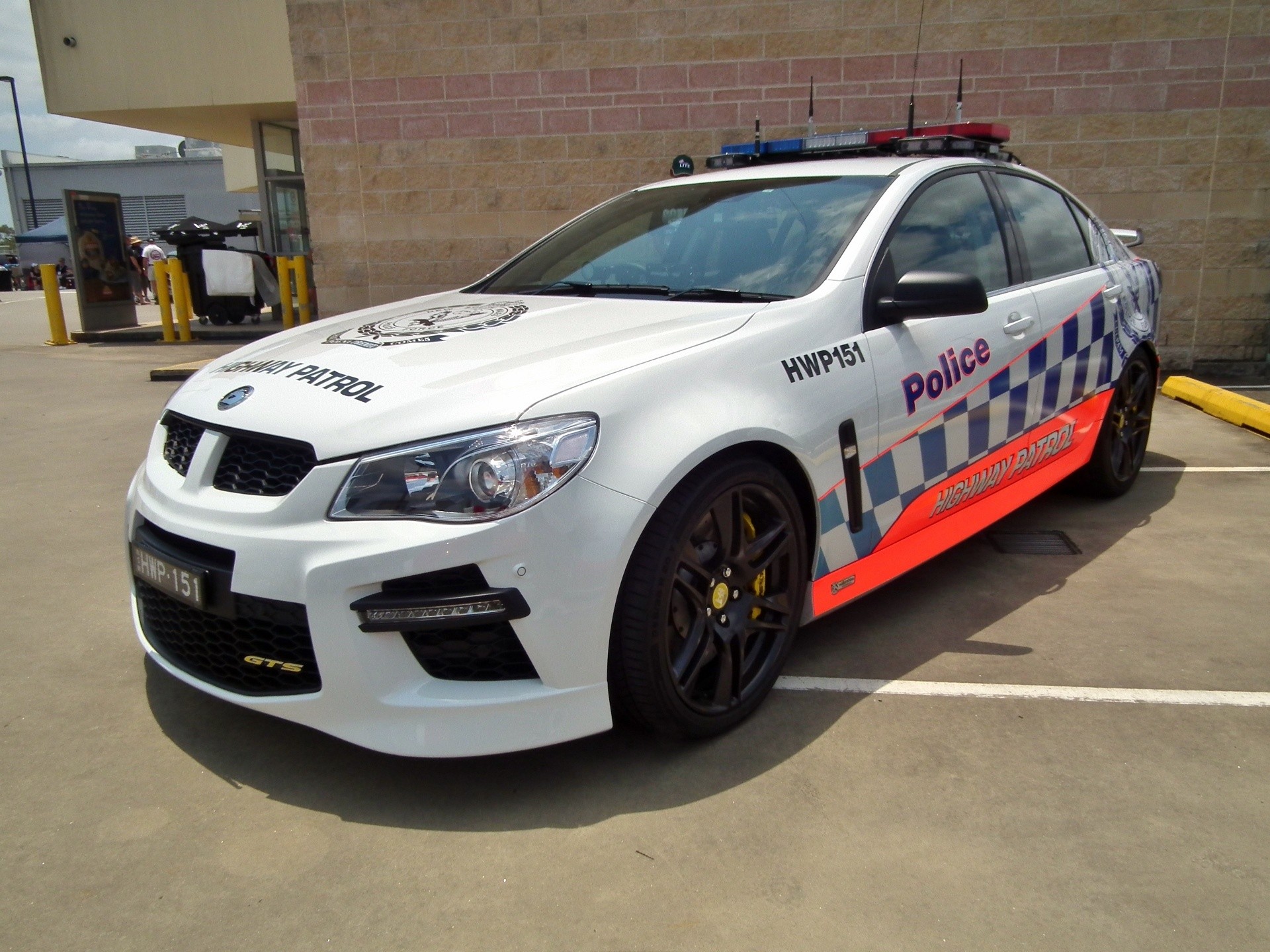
(0, 75), (39, 228)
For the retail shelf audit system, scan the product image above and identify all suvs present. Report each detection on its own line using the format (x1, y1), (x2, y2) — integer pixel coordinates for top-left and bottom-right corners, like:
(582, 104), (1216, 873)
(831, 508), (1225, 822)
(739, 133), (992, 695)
(0, 253), (20, 269)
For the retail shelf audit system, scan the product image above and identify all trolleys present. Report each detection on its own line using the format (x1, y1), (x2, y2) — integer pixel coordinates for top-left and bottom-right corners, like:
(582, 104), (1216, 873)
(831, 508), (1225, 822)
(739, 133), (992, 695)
(165, 232), (270, 326)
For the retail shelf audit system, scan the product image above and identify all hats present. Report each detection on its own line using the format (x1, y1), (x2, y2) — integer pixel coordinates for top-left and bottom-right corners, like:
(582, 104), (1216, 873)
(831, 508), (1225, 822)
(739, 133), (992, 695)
(31, 264), (38, 267)
(58, 257), (65, 261)
(147, 238), (154, 242)
(130, 236), (141, 244)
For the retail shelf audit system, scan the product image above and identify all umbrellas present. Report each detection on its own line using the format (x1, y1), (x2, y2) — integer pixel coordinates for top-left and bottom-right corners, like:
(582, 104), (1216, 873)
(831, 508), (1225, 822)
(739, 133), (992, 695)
(14, 215), (69, 243)
(224, 219), (261, 251)
(153, 216), (239, 237)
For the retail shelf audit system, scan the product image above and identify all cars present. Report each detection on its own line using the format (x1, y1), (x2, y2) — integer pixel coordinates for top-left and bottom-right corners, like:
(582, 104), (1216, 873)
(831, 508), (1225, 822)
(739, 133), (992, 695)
(129, 0), (1162, 765)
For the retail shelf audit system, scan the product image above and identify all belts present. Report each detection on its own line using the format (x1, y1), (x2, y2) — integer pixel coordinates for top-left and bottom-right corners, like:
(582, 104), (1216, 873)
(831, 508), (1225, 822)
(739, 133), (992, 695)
(149, 264), (153, 266)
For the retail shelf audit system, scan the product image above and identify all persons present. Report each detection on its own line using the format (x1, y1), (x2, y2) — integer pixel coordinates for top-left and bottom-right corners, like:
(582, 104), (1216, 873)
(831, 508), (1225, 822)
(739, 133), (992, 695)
(56, 258), (68, 289)
(141, 237), (167, 304)
(125, 234), (152, 305)
(29, 263), (43, 290)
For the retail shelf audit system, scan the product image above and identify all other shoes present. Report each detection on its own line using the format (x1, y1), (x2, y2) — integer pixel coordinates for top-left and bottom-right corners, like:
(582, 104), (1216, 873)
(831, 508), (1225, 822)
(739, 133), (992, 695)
(134, 296), (140, 303)
(144, 298), (151, 302)
(154, 300), (159, 304)
(141, 301), (148, 304)
